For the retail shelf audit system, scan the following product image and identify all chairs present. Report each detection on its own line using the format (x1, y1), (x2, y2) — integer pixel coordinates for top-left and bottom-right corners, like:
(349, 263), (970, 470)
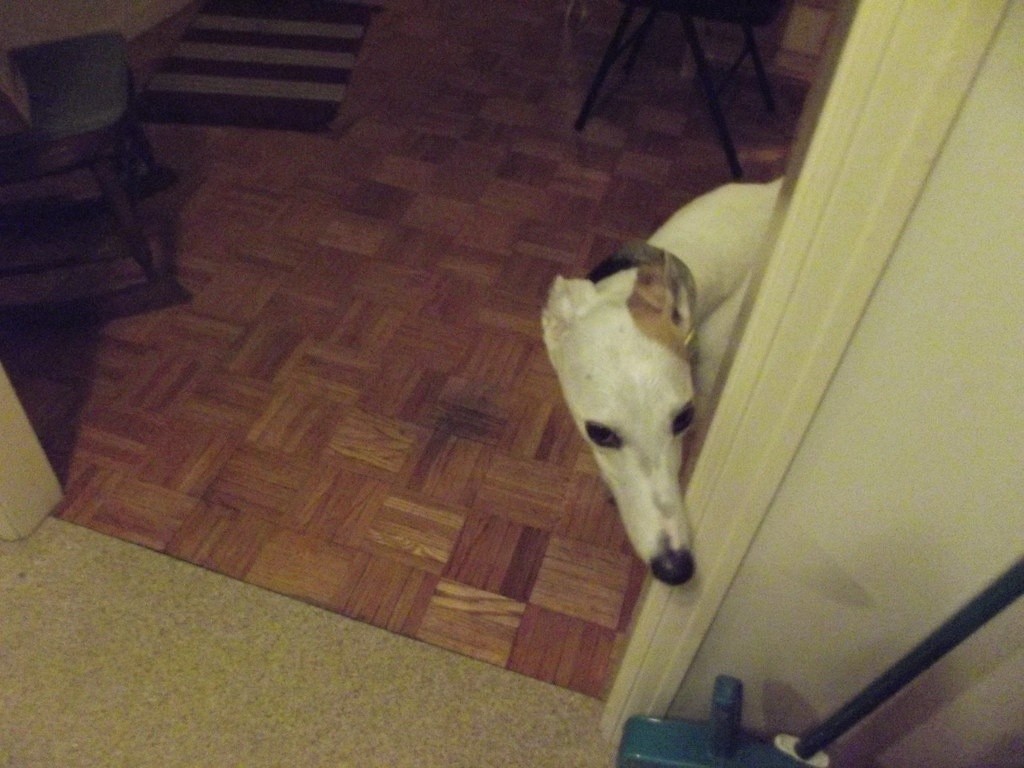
(0, 30), (163, 297)
(574, 0), (781, 180)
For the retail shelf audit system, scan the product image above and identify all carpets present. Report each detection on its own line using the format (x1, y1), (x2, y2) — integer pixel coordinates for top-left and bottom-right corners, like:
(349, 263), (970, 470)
(139, 0), (373, 133)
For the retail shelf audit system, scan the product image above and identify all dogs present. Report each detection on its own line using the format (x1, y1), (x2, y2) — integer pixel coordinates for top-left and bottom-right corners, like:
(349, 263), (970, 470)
(542, 176), (785, 587)
